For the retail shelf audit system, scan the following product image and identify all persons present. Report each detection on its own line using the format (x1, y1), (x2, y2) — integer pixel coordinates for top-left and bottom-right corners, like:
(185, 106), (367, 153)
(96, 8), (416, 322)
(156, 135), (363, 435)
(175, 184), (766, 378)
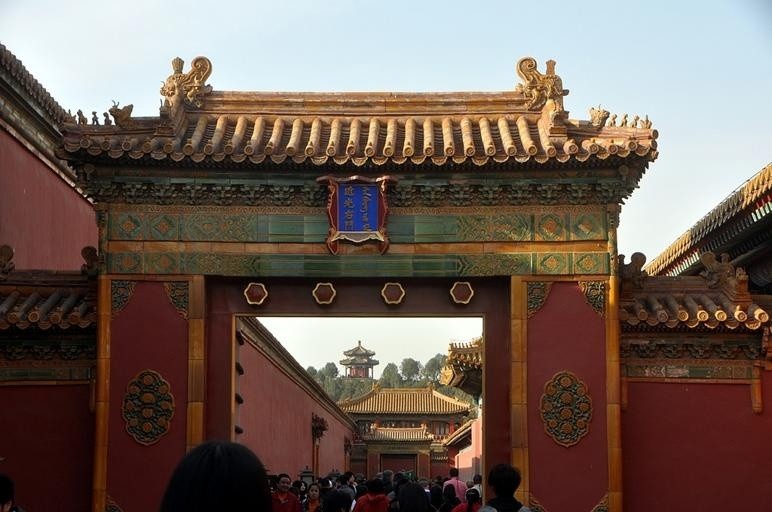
(0, 475), (24, 512)
(159, 439), (273, 511)
(268, 464), (530, 512)
